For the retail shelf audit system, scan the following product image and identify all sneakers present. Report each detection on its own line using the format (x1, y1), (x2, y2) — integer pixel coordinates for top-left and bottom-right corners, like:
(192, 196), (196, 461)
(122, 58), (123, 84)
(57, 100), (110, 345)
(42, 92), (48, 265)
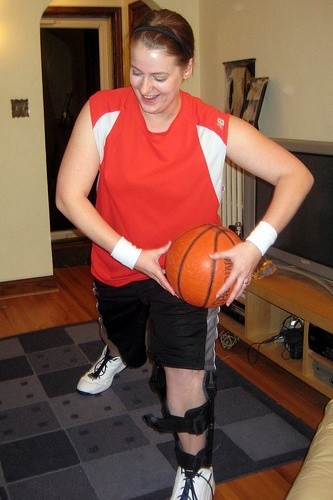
(169, 464), (215, 500)
(76, 344), (127, 394)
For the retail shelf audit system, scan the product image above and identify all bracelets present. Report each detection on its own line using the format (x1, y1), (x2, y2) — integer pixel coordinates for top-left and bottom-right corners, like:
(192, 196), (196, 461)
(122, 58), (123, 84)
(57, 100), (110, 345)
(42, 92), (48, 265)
(246, 221), (278, 256)
(110, 236), (142, 270)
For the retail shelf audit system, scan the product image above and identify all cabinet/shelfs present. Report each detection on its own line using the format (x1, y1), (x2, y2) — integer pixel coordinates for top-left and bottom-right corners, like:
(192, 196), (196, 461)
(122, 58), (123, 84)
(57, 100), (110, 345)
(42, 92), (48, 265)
(218, 269), (333, 400)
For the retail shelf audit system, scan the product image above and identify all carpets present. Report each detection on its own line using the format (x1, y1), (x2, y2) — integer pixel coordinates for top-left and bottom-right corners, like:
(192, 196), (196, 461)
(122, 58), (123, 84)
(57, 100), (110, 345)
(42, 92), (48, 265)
(0, 319), (317, 500)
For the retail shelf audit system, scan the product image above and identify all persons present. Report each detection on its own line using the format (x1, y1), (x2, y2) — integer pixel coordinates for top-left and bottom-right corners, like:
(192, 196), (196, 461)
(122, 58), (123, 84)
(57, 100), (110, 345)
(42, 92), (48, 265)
(56, 9), (314, 500)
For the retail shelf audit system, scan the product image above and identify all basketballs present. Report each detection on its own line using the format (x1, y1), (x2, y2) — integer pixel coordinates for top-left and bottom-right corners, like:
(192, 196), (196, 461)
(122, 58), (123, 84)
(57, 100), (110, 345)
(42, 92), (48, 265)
(166, 224), (244, 308)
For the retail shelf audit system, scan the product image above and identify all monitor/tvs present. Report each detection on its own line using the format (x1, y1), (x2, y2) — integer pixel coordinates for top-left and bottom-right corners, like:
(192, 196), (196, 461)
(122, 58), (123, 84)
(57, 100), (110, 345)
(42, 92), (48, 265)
(242, 137), (333, 284)
